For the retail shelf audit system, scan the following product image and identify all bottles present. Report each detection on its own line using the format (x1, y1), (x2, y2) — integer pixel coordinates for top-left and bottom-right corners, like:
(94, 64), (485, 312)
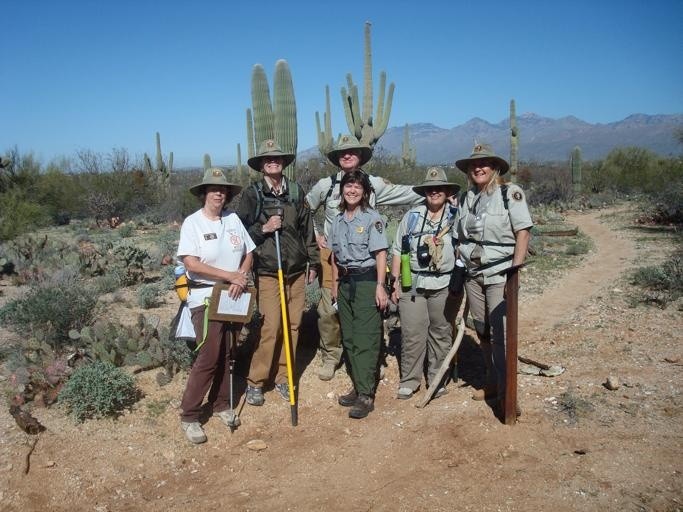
(448, 259), (465, 295)
(401, 250), (412, 288)
(174, 266), (189, 302)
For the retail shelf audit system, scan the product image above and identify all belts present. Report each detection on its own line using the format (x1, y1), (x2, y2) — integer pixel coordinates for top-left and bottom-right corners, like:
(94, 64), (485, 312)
(338, 266), (369, 276)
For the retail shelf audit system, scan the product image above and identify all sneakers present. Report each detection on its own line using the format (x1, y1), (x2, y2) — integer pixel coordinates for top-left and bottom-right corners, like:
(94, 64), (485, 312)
(245, 383), (264, 406)
(500, 398), (520, 416)
(210, 407), (241, 428)
(273, 381), (291, 401)
(339, 388), (374, 418)
(473, 384), (497, 401)
(318, 362), (336, 380)
(398, 387), (415, 399)
(435, 385), (448, 397)
(180, 421), (207, 443)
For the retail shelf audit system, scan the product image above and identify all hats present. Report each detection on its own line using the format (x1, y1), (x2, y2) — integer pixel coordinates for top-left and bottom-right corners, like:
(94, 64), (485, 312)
(412, 166), (461, 197)
(247, 140), (295, 172)
(327, 136), (373, 167)
(456, 146), (509, 177)
(190, 168), (242, 197)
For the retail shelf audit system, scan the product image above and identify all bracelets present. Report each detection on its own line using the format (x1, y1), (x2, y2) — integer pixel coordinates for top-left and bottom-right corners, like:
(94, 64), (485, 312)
(377, 282), (385, 286)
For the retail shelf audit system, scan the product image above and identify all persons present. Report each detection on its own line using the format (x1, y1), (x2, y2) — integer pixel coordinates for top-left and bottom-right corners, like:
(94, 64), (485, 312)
(388, 165), (458, 400)
(236, 141), (320, 406)
(303, 135), (457, 381)
(177, 168), (257, 445)
(452, 145), (533, 416)
(327, 170), (390, 418)
